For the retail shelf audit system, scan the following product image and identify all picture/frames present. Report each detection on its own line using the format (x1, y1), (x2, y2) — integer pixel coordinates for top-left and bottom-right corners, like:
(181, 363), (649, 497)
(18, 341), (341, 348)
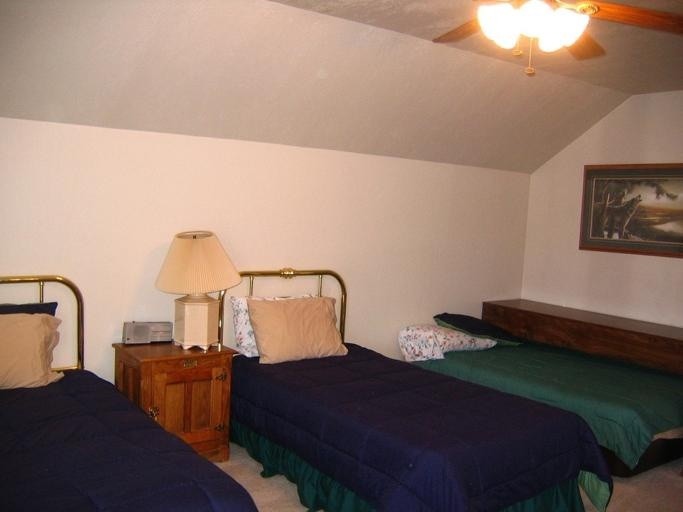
(579, 164), (683, 260)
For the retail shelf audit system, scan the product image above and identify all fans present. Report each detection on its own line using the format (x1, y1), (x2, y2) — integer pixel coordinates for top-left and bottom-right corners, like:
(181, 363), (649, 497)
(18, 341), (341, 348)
(432, 1), (683, 62)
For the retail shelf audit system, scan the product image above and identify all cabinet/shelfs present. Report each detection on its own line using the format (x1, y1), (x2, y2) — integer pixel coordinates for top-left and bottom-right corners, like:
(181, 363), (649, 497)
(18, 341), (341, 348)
(481, 297), (683, 378)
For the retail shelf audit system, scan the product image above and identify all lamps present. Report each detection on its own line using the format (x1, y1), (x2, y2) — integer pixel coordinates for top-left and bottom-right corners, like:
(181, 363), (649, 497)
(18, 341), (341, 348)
(474, 0), (599, 75)
(153, 229), (244, 352)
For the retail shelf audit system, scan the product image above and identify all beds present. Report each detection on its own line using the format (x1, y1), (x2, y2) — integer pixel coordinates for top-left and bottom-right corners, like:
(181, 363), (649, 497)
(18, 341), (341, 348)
(0, 274), (258, 512)
(398, 312), (683, 478)
(217, 268), (615, 511)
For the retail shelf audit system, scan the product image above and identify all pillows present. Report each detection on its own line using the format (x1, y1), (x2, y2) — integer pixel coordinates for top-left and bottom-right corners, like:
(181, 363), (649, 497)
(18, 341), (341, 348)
(0, 312), (65, 390)
(0, 302), (58, 317)
(403, 322), (498, 354)
(397, 329), (446, 363)
(433, 313), (526, 347)
(246, 296), (349, 365)
(229, 294), (317, 359)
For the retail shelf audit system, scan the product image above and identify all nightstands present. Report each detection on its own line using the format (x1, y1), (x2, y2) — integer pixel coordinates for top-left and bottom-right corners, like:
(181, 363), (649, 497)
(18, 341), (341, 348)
(112, 340), (239, 462)
